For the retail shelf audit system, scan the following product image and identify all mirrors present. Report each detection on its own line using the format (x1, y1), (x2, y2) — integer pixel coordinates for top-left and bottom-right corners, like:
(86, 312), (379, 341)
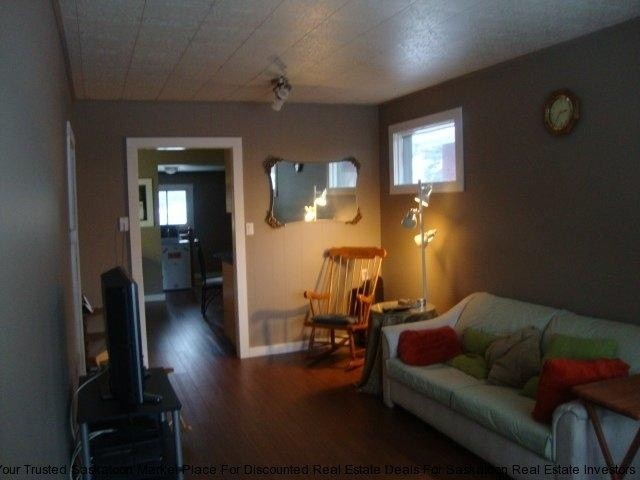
(262, 153), (364, 230)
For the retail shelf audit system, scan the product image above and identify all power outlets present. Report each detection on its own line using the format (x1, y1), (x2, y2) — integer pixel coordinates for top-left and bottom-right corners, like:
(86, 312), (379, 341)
(245, 222), (254, 235)
(119, 216), (129, 233)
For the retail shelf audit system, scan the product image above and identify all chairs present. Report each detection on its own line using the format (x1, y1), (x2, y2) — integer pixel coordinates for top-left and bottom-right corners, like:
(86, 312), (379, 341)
(302, 246), (387, 370)
(194, 238), (223, 321)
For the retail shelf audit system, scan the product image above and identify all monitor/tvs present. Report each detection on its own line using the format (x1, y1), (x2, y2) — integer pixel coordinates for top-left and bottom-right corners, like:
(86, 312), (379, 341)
(100, 266), (144, 407)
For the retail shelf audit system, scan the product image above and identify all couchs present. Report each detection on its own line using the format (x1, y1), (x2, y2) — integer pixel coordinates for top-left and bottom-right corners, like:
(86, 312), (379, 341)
(380, 291), (639, 478)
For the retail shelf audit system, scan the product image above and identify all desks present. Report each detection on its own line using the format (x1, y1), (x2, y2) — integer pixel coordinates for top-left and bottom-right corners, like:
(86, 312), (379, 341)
(367, 298), (438, 392)
(79, 366), (186, 480)
(570, 373), (639, 480)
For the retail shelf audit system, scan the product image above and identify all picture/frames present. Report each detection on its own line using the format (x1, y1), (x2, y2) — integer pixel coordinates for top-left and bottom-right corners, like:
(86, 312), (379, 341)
(138, 177), (155, 228)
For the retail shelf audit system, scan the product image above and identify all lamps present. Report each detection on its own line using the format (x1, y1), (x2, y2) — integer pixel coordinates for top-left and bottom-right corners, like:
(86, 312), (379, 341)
(301, 184), (328, 223)
(269, 74), (292, 114)
(398, 178), (439, 309)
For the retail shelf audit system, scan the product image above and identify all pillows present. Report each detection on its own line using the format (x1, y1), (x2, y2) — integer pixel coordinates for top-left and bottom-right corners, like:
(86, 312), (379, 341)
(484, 324), (542, 387)
(445, 326), (504, 379)
(397, 324), (462, 366)
(517, 333), (619, 399)
(531, 358), (631, 424)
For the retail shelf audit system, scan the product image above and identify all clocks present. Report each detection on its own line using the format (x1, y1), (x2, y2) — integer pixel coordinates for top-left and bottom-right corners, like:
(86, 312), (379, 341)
(541, 88), (581, 137)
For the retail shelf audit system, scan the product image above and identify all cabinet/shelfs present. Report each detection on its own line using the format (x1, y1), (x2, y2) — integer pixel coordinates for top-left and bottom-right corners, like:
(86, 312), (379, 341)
(221, 258), (235, 346)
